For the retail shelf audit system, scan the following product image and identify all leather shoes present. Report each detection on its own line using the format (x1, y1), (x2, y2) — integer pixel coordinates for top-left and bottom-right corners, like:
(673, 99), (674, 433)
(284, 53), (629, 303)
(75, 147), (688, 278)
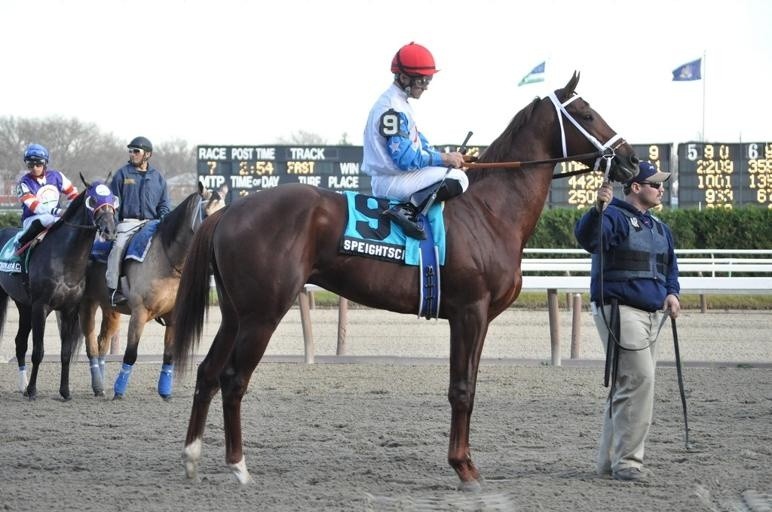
(615, 467), (650, 480)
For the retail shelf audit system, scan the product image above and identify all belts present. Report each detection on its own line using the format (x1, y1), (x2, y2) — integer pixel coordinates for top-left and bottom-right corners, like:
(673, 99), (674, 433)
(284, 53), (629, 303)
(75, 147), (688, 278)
(596, 297), (656, 313)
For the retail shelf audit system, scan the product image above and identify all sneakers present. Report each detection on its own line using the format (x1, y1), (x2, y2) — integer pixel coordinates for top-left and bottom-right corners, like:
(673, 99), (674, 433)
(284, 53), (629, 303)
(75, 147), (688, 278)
(13, 238), (25, 258)
(108, 288), (128, 306)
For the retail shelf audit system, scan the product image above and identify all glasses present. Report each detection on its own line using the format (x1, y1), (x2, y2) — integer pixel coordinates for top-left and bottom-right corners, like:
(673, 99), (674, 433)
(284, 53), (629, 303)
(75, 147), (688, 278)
(128, 149), (140, 154)
(25, 161), (42, 169)
(639, 182), (661, 189)
(413, 78), (431, 90)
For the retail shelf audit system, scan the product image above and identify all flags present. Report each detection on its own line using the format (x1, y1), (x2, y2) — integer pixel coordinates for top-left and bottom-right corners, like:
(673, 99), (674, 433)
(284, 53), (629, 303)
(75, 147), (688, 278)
(673, 58), (702, 81)
(519, 62), (545, 87)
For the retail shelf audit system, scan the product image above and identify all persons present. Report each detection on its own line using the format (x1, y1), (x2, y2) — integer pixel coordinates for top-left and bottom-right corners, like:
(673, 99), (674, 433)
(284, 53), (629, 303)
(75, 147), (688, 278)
(105, 137), (172, 304)
(360, 42), (469, 241)
(13, 143), (80, 250)
(575, 162), (680, 483)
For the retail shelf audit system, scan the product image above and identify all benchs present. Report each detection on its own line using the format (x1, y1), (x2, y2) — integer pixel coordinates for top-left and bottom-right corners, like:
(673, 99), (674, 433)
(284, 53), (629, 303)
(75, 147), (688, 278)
(521, 248), (772, 313)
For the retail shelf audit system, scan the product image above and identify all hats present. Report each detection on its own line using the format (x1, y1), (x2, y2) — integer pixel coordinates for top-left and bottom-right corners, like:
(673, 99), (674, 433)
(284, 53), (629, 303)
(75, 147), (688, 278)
(127, 137), (152, 152)
(621, 161), (672, 184)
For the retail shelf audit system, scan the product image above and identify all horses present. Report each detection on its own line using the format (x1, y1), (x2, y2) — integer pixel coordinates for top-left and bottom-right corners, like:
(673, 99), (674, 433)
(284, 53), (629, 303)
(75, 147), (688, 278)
(0, 170), (120, 403)
(54, 179), (231, 402)
(166, 69), (643, 490)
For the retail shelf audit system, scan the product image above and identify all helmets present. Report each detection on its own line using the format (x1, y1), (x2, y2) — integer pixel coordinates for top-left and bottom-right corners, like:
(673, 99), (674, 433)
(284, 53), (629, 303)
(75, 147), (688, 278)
(391, 41), (440, 76)
(23, 144), (48, 162)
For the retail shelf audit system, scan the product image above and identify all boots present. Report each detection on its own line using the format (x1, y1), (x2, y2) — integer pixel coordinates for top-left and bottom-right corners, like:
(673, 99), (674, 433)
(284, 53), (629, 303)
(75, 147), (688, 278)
(382, 180), (450, 240)
